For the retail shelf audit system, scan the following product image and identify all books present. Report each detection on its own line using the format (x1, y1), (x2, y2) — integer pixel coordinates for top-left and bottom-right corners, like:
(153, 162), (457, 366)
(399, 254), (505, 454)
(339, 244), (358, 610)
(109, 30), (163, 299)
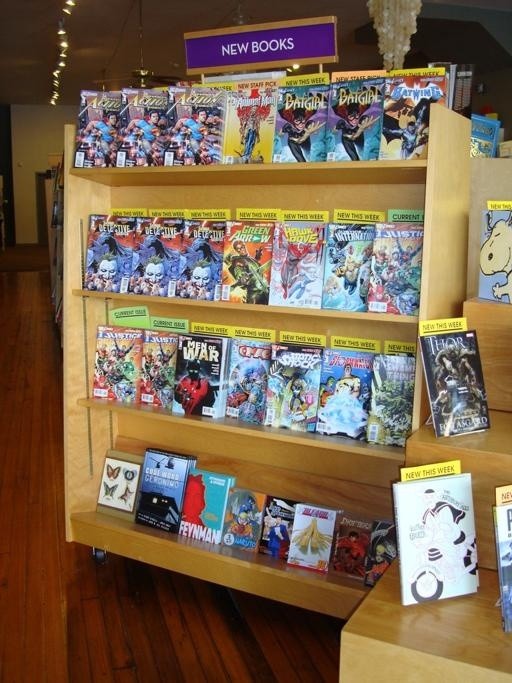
(84, 208), (424, 317)
(477, 209), (511, 303)
(91, 325), (417, 449)
(492, 501), (512, 634)
(96, 447), (399, 585)
(392, 471), (480, 607)
(72, 66), (451, 168)
(418, 328), (490, 437)
(469, 114), (501, 158)
(497, 139), (511, 157)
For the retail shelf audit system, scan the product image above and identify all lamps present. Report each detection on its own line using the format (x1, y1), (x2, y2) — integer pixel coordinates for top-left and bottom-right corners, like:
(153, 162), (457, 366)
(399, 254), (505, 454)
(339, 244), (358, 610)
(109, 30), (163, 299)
(366, 0), (423, 71)
(130, 0), (152, 89)
(48, 0), (77, 106)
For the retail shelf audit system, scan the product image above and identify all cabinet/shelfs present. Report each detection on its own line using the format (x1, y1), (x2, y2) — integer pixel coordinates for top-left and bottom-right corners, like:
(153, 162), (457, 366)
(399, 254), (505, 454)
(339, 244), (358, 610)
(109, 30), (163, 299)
(337, 294), (510, 683)
(55, 60), (470, 625)
